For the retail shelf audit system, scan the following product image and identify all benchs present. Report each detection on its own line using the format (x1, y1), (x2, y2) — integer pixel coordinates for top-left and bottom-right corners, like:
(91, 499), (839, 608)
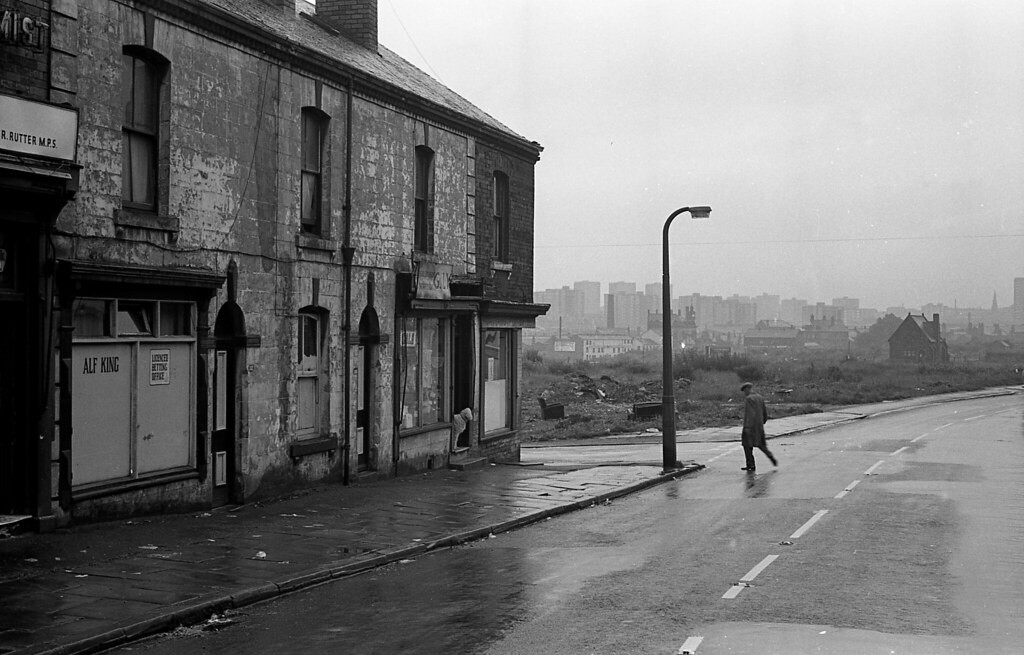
(634, 401), (680, 421)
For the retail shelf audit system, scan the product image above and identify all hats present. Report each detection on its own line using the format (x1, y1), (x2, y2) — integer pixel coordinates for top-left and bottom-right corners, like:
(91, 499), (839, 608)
(741, 382), (753, 391)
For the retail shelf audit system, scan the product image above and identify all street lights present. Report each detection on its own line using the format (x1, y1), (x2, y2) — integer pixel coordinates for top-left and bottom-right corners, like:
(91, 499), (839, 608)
(663, 206), (714, 470)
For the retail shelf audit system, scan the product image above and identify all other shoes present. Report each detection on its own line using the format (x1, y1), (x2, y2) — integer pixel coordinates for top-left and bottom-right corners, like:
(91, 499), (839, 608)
(741, 466), (756, 471)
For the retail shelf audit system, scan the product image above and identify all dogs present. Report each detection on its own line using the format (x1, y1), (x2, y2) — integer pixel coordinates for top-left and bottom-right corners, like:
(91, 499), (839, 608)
(454, 408), (472, 450)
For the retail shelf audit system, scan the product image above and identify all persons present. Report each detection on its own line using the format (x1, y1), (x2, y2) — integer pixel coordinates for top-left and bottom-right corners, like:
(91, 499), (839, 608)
(740, 383), (779, 471)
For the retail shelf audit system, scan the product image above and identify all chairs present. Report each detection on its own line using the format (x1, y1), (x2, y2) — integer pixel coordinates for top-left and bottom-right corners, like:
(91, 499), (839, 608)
(536, 397), (564, 421)
